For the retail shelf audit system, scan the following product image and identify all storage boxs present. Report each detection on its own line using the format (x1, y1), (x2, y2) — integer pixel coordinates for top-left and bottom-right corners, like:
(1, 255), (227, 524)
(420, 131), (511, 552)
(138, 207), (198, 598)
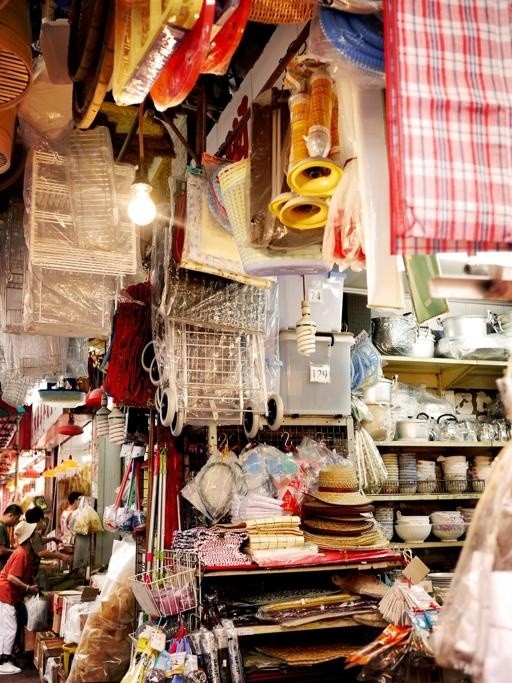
(279, 330), (358, 417)
(275, 265), (347, 330)
(25, 574), (98, 682)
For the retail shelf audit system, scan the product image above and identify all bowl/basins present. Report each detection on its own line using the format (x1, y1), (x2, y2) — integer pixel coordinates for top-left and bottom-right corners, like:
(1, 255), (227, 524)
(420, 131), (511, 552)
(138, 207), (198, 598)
(464, 262), (503, 278)
(376, 452), (493, 493)
(374, 507), (477, 543)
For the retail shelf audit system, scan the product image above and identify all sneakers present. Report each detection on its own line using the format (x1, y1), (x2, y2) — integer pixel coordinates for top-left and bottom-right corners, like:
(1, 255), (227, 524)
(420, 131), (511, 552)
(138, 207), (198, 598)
(0, 662), (21, 675)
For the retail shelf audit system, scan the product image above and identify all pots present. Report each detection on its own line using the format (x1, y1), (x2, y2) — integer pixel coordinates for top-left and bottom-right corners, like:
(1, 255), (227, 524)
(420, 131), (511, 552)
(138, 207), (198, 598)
(397, 411), (465, 442)
(358, 372), (396, 406)
(370, 307), (512, 361)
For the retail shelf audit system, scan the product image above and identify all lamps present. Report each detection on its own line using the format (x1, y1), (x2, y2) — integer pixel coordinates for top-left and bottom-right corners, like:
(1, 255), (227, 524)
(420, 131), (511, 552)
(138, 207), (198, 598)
(21, 389), (129, 484)
(297, 275), (318, 356)
(127, 105), (156, 227)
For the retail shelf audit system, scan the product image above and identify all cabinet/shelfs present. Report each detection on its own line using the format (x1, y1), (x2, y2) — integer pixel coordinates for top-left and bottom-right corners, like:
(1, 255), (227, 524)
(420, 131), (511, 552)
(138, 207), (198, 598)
(369, 354), (512, 554)
(170, 539), (413, 681)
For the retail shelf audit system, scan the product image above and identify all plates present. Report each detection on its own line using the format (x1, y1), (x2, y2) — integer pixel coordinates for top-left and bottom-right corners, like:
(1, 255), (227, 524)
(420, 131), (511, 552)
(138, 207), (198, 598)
(392, 573), (456, 591)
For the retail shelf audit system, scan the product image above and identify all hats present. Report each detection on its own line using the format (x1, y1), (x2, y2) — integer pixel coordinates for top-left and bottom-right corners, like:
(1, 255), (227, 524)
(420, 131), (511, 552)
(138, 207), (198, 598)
(15, 522), (38, 546)
(301, 464), (388, 551)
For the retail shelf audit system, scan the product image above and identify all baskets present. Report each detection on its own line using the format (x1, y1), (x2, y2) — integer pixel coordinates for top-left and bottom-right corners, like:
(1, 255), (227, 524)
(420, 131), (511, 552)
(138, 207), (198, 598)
(226, 0), (315, 25)
(218, 158), (334, 277)
(128, 565), (198, 619)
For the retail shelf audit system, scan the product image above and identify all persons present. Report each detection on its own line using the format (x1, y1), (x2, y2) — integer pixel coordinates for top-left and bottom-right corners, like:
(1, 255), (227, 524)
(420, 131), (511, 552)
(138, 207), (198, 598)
(428, 357), (512, 683)
(0, 491), (85, 677)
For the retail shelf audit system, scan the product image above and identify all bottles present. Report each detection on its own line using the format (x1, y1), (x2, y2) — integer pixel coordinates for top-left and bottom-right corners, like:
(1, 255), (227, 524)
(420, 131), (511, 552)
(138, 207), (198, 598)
(56, 540), (65, 552)
